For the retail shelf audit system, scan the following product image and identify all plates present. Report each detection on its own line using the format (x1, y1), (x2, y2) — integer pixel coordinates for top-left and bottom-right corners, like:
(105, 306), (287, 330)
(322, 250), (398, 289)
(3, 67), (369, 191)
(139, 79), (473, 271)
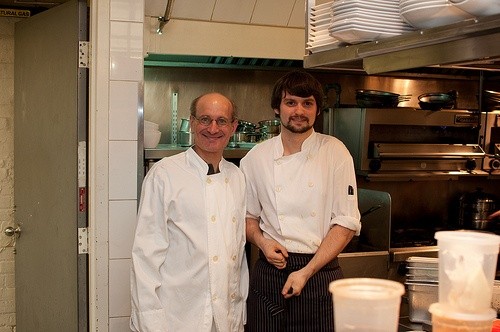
(238, 143), (256, 147)
(327, 0), (417, 45)
(305, 2), (339, 53)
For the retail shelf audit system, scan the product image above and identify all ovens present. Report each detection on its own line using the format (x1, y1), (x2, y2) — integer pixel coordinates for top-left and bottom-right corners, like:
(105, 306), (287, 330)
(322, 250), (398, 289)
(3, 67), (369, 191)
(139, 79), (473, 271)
(330, 108), (500, 179)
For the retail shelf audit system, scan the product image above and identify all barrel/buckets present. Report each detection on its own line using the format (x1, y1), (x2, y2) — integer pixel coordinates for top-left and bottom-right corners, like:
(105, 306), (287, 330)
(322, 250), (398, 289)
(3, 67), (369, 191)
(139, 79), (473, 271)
(428, 231), (500, 332)
(329, 278), (405, 331)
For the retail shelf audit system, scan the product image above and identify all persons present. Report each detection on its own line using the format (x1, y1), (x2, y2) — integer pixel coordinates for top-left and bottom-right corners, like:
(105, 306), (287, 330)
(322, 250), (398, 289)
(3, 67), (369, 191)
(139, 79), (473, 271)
(129, 92), (250, 332)
(239, 70), (362, 332)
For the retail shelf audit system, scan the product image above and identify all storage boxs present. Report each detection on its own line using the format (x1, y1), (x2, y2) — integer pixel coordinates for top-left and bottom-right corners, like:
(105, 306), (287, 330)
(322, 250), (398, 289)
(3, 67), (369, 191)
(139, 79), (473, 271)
(329, 277), (406, 332)
(434, 230), (500, 314)
(428, 303), (497, 332)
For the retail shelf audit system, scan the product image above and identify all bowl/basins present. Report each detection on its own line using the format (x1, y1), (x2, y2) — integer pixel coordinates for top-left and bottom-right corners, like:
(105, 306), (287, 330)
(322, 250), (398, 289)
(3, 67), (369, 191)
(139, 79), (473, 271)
(143, 119), (161, 148)
(179, 119), (197, 147)
(400, 0), (463, 29)
(449, 0), (500, 17)
(262, 134), (280, 141)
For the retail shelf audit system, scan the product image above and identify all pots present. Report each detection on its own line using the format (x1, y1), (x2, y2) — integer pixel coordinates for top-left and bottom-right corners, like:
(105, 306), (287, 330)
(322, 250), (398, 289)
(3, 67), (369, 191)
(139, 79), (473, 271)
(355, 89), (413, 107)
(228, 120), (262, 148)
(249, 120), (282, 134)
(418, 89), (458, 110)
(458, 188), (496, 229)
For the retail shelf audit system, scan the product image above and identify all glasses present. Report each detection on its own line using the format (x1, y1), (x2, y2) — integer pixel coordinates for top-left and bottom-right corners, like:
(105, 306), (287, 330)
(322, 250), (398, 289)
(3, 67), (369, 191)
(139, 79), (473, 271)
(191, 115), (238, 128)
